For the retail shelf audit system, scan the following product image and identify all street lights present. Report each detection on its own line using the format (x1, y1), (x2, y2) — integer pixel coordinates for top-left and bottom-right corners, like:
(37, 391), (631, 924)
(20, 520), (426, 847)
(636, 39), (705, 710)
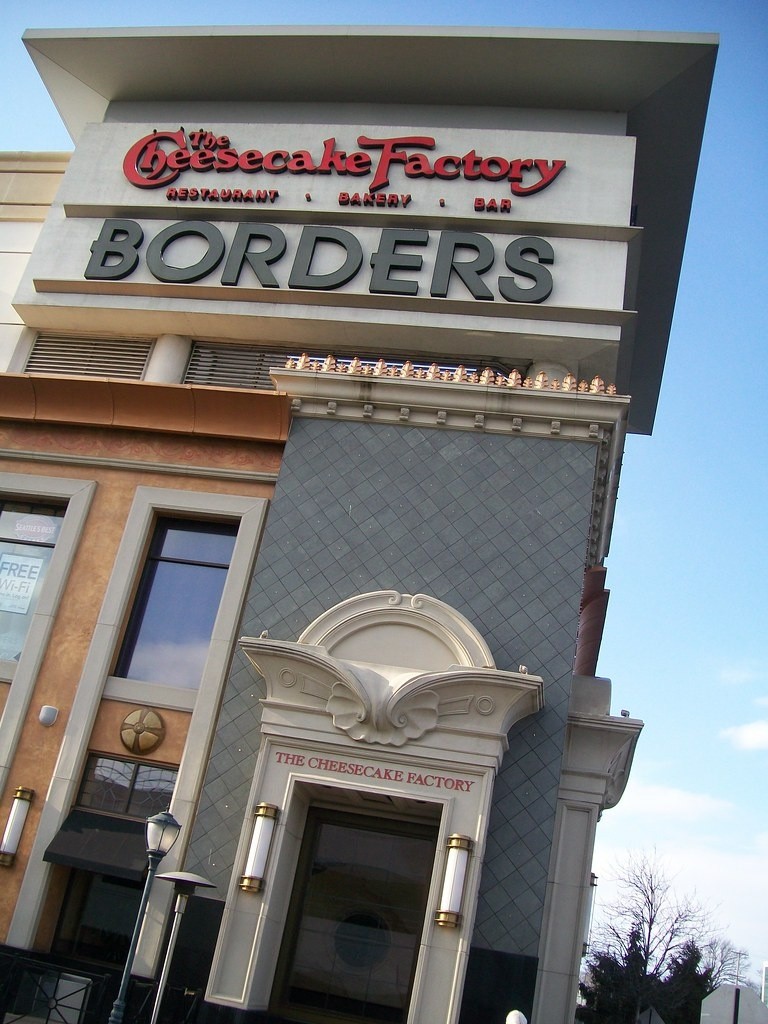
(731, 951), (748, 985)
(108, 802), (183, 1024)
